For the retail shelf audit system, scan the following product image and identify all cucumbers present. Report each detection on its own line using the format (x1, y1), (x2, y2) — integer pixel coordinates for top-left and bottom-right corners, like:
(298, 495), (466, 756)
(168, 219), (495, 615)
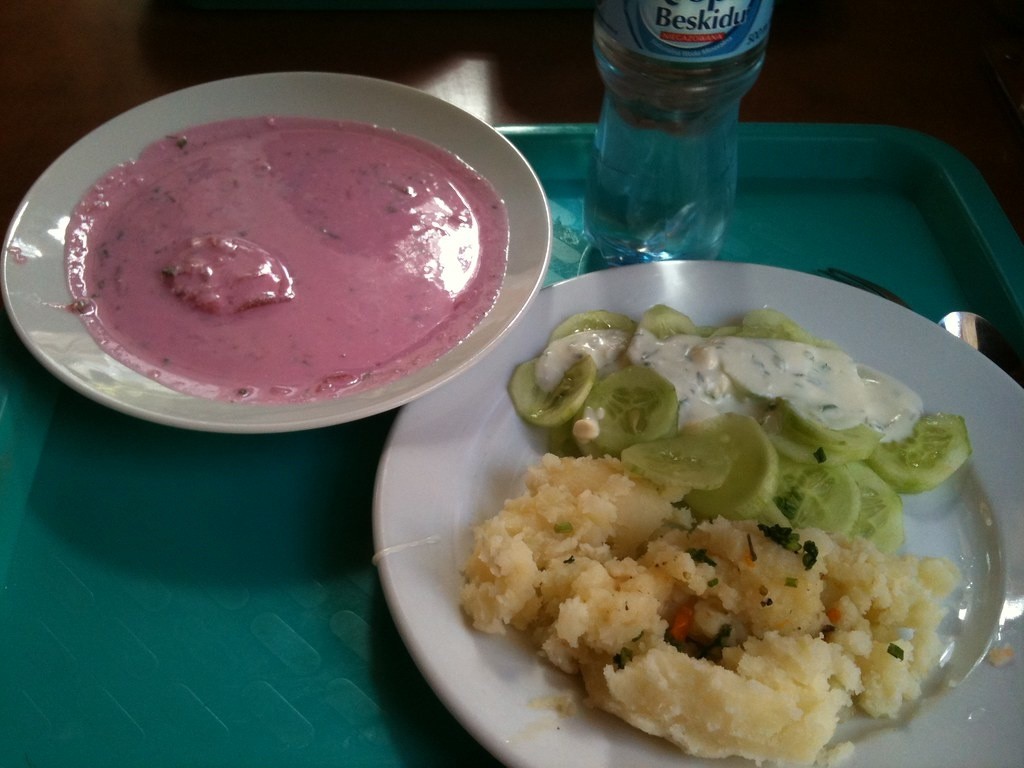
(508, 302), (969, 540)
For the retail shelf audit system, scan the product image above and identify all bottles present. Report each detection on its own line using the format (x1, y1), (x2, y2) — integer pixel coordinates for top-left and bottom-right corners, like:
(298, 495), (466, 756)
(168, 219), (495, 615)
(583, 0), (774, 266)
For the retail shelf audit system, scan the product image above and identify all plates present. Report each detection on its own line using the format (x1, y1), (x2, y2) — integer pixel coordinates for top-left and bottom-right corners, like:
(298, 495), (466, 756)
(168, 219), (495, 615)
(372, 259), (1024, 768)
(0, 72), (553, 434)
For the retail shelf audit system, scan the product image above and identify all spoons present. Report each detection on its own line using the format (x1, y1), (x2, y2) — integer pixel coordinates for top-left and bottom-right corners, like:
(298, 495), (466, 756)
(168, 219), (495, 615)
(936, 310), (1024, 389)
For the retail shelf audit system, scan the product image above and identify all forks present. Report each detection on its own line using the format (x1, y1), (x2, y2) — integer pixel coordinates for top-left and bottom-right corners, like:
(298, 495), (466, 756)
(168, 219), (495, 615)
(810, 267), (911, 310)
